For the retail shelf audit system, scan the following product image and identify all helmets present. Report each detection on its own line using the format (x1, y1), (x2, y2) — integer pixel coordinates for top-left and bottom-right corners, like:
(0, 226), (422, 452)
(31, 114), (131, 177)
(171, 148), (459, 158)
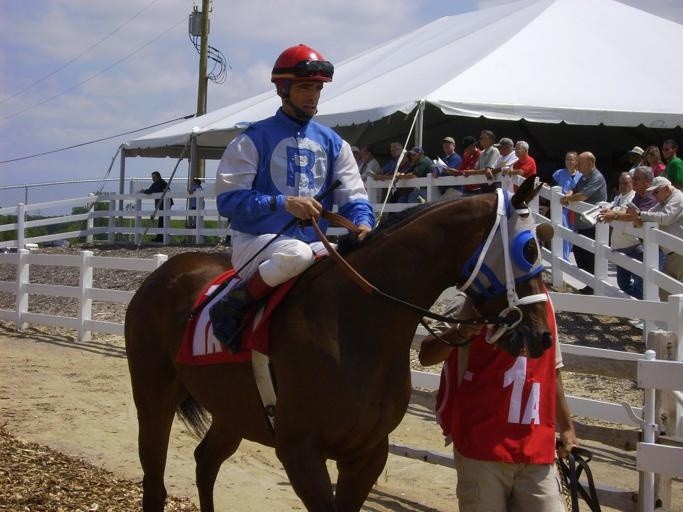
(271, 44), (332, 82)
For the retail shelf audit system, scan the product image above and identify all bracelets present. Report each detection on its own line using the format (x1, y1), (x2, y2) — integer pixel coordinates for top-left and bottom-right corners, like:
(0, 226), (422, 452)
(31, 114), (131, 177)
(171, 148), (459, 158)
(457, 323), (467, 340)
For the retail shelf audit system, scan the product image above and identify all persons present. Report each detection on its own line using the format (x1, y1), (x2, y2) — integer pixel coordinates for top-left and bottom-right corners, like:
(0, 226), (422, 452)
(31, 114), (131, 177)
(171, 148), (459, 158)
(189, 179), (205, 227)
(208, 44), (374, 355)
(351, 131), (537, 215)
(419, 281), (578, 512)
(596, 138), (683, 298)
(548, 151), (583, 263)
(560, 152), (607, 295)
(140, 171), (174, 242)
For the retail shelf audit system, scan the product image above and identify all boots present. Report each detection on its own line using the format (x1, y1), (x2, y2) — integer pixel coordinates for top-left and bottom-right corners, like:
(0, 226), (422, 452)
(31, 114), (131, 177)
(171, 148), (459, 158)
(208, 279), (254, 355)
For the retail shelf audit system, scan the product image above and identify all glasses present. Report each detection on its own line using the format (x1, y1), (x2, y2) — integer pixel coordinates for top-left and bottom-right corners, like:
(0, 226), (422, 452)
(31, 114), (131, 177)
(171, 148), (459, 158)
(294, 60), (334, 77)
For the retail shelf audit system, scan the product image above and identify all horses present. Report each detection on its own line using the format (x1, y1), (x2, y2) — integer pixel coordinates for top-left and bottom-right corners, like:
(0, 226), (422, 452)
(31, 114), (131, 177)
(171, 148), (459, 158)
(123, 175), (553, 512)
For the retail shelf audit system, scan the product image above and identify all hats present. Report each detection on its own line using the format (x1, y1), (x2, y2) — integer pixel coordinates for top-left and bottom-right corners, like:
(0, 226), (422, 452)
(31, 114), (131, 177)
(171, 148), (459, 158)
(645, 176), (671, 192)
(442, 136), (456, 143)
(408, 147), (424, 155)
(460, 136), (476, 150)
(351, 146), (359, 151)
(628, 145), (645, 155)
(493, 138), (513, 147)
